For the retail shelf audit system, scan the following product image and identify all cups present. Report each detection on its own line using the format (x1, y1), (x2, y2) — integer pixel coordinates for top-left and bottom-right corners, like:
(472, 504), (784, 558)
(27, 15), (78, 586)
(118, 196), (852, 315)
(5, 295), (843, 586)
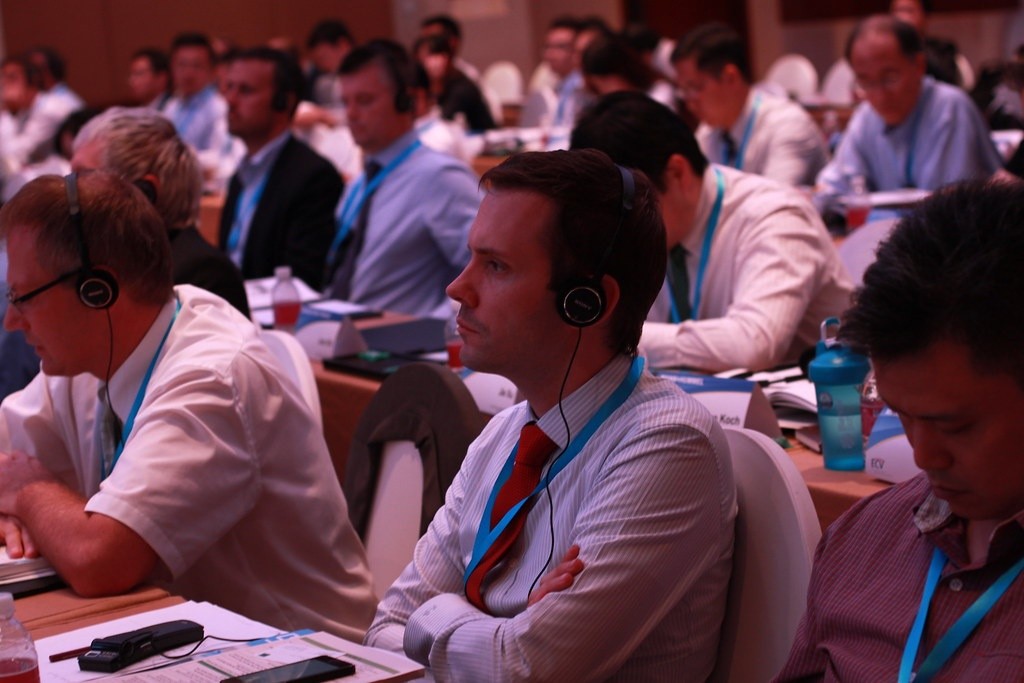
(502, 104), (525, 128)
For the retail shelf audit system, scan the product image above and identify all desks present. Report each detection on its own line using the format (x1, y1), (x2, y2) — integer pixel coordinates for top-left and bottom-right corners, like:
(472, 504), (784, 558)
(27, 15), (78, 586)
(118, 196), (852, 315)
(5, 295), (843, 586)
(0, 150), (1024, 683)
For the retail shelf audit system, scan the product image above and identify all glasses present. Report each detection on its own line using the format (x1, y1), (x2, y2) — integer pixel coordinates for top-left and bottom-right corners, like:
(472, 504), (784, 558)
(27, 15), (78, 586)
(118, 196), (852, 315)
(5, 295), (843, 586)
(5, 260), (115, 316)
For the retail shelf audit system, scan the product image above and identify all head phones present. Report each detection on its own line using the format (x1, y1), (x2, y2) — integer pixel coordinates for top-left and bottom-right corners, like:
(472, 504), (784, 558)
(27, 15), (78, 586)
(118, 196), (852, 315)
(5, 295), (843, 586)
(732, 345), (817, 388)
(132, 178), (157, 205)
(378, 42), (413, 112)
(65, 172), (121, 311)
(272, 58), (291, 110)
(556, 165), (635, 329)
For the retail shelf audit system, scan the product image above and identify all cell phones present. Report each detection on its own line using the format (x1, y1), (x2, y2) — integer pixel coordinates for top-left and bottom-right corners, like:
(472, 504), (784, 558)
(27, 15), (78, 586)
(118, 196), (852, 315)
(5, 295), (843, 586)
(219, 655), (356, 683)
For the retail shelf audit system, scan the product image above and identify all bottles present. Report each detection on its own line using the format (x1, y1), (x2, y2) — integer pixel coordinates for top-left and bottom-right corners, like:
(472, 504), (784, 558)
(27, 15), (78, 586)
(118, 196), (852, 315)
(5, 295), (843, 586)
(270, 267), (300, 335)
(847, 175), (868, 230)
(808, 317), (871, 472)
(0, 591), (41, 683)
(444, 301), (467, 373)
(861, 364), (887, 460)
(538, 114), (555, 151)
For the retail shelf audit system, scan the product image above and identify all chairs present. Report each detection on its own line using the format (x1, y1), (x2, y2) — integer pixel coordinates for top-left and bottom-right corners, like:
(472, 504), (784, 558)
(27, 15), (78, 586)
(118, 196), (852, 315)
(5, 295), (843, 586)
(715, 421), (827, 683)
(338, 354), (486, 548)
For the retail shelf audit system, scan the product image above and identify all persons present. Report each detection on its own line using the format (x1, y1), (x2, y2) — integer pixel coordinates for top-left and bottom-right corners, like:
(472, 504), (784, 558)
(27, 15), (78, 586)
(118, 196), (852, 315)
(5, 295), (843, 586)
(0, 0), (1024, 683)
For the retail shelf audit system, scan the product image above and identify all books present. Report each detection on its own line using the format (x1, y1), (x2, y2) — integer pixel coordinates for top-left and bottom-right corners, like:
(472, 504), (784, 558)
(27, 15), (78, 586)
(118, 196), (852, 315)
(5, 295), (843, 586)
(0, 547), (68, 601)
(91, 628), (426, 683)
(765, 379), (819, 415)
(288, 298), (372, 332)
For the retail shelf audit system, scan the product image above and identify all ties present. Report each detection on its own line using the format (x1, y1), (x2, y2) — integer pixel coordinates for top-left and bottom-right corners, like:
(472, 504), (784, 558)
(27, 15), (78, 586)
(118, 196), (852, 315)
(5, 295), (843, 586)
(665, 238), (690, 324)
(329, 160), (382, 301)
(463, 422), (559, 614)
(99, 402), (119, 478)
(715, 128), (740, 166)
